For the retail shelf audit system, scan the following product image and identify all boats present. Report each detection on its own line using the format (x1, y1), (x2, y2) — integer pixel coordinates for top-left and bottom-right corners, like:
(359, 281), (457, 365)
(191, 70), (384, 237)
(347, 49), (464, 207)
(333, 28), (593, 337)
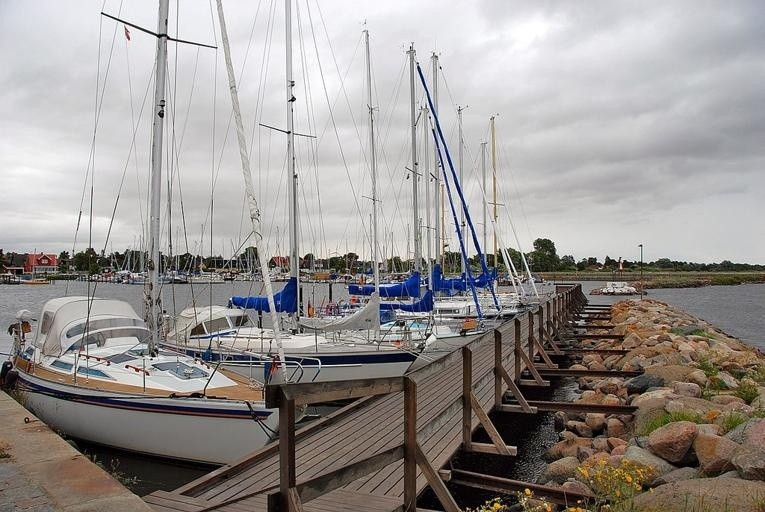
(90, 234), (165, 284)
(286, 1), (544, 341)
(150, 0), (440, 385)
(165, 224), (227, 285)
(0, 1), (322, 466)
